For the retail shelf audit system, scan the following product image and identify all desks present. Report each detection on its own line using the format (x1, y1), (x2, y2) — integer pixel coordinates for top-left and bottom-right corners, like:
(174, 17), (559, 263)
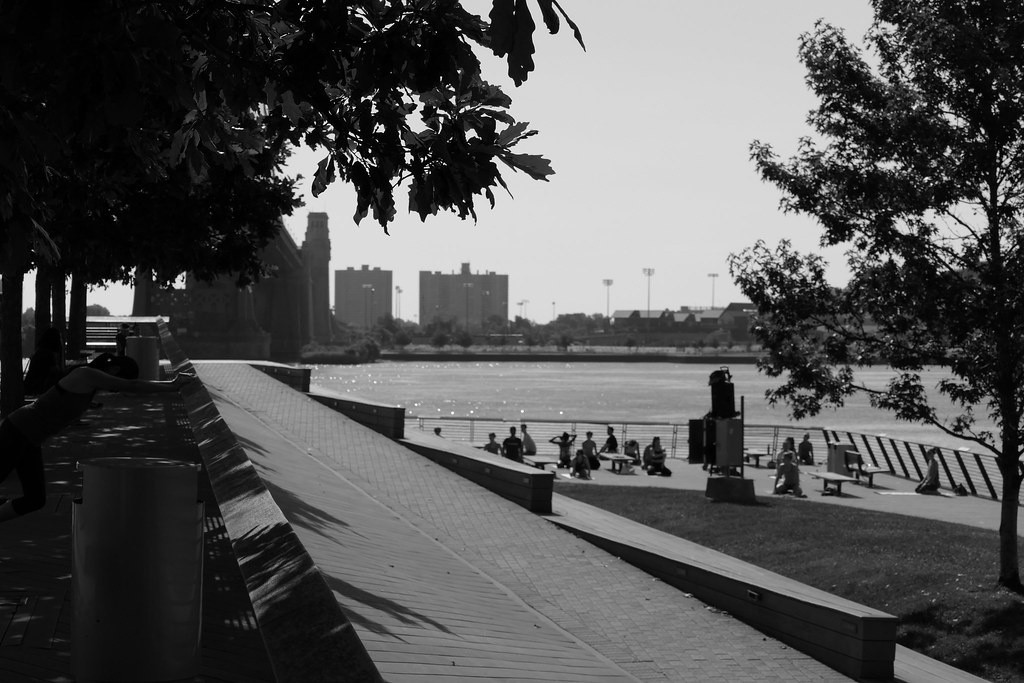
(807, 472), (861, 496)
(524, 455), (558, 470)
(599, 452), (635, 473)
(744, 449), (766, 467)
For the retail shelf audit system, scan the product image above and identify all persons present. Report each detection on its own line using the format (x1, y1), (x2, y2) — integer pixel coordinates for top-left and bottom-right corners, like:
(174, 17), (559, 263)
(643, 437), (671, 476)
(625, 440), (641, 465)
(569, 449), (591, 479)
(0, 353), (195, 523)
(25, 327), (104, 427)
(583, 432), (597, 456)
(521, 424), (536, 455)
(484, 426), (523, 462)
(601, 427), (618, 452)
(799, 434), (814, 465)
(777, 437), (797, 465)
(550, 432), (577, 468)
(774, 452), (802, 496)
(915, 449), (940, 495)
(435, 428), (441, 435)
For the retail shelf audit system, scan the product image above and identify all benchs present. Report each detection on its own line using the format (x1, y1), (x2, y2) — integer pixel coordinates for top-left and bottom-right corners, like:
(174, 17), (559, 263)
(843, 450), (891, 488)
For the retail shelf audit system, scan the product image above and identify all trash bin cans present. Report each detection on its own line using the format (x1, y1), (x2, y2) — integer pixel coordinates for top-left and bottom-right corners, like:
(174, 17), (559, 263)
(827, 442), (855, 478)
(125, 337), (160, 380)
(70, 456), (206, 683)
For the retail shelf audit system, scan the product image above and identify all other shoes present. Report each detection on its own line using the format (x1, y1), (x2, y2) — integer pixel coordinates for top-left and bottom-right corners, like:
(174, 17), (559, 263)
(89, 402), (103, 410)
(70, 422), (92, 429)
